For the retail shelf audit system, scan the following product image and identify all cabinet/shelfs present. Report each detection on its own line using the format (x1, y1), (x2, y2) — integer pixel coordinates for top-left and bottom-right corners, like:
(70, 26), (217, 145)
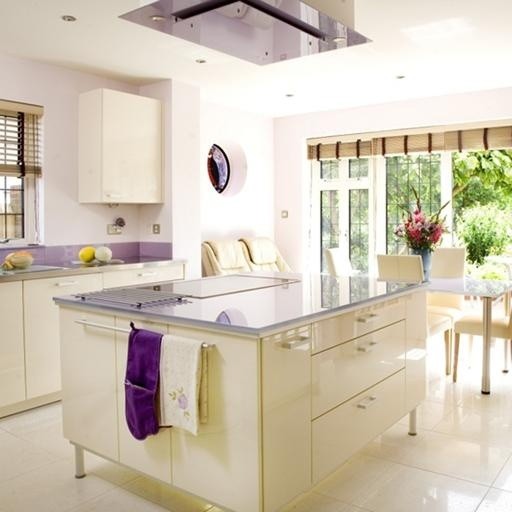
(0, 262), (188, 418)
(59, 289), (427, 512)
(79, 88), (165, 204)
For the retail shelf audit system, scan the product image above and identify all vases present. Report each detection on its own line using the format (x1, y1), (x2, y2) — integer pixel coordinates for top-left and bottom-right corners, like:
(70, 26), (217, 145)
(412, 249), (431, 282)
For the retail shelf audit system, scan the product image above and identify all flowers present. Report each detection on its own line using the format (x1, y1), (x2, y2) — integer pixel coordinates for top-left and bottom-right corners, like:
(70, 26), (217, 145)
(392, 181), (470, 253)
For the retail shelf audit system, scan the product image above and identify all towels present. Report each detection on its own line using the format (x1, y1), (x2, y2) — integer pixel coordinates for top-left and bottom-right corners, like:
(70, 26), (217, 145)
(157, 334), (209, 437)
(124, 329), (164, 440)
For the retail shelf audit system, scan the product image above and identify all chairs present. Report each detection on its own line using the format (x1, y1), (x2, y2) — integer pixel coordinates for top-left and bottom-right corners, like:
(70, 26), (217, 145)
(326, 247), (352, 276)
(201, 240), (251, 277)
(377, 255), (453, 375)
(453, 308), (512, 382)
(431, 248), (465, 374)
(238, 237), (294, 273)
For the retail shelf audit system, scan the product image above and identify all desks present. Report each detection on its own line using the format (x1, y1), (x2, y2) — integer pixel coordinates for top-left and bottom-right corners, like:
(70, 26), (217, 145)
(423, 275), (512, 394)
